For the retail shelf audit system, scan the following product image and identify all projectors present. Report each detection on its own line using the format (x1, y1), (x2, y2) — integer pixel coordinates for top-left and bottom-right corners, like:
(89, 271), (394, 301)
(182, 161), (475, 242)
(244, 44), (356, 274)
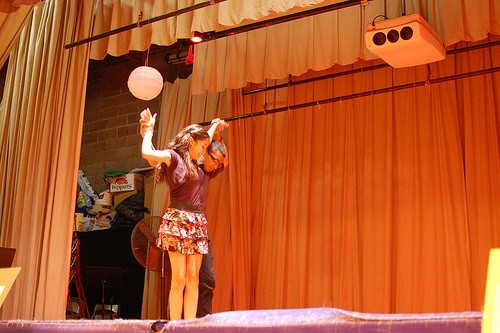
(366, 12), (446, 69)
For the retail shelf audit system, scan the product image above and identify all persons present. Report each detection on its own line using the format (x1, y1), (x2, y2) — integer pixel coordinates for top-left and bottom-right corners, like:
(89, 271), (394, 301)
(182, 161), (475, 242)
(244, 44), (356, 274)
(138, 119), (229, 319)
(139, 107), (229, 321)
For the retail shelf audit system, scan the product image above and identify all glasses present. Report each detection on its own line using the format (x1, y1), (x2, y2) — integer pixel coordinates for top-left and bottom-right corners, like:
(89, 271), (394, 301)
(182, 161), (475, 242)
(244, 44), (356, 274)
(208, 153), (223, 168)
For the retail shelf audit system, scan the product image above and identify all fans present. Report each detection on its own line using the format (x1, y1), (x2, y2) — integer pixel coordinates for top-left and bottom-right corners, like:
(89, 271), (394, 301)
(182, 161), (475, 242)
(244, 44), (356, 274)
(131, 216), (169, 319)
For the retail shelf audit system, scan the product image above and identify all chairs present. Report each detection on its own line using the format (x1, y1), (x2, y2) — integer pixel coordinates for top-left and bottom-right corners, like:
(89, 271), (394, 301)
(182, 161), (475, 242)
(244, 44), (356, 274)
(69, 231), (91, 320)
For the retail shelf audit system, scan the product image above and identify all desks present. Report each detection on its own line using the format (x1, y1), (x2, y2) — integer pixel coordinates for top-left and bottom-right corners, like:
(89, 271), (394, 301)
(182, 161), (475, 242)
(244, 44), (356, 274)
(70, 224), (145, 319)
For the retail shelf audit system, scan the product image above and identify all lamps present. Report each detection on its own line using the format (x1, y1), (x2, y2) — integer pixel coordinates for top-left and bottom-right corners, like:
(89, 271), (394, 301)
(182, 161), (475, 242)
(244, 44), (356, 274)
(191, 31), (204, 42)
(126, 46), (164, 101)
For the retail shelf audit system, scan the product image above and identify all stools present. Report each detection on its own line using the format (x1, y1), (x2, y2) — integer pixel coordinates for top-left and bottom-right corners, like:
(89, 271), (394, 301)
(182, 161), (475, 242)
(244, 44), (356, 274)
(86, 266), (128, 320)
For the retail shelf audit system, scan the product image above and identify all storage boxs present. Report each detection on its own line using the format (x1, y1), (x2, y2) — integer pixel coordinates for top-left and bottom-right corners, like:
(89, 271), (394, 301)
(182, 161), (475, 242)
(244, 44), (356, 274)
(114, 190), (138, 208)
(109, 173), (143, 192)
